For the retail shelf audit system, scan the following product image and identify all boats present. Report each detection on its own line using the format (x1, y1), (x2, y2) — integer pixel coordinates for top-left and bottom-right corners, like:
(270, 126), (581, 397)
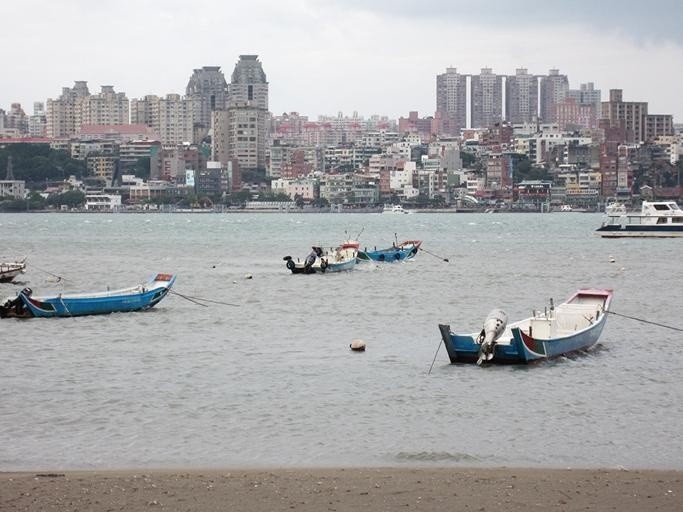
(14, 273), (177, 318)
(0, 255), (30, 283)
(282, 240), (360, 276)
(593, 198), (682, 238)
(356, 238), (422, 263)
(437, 287), (613, 366)
